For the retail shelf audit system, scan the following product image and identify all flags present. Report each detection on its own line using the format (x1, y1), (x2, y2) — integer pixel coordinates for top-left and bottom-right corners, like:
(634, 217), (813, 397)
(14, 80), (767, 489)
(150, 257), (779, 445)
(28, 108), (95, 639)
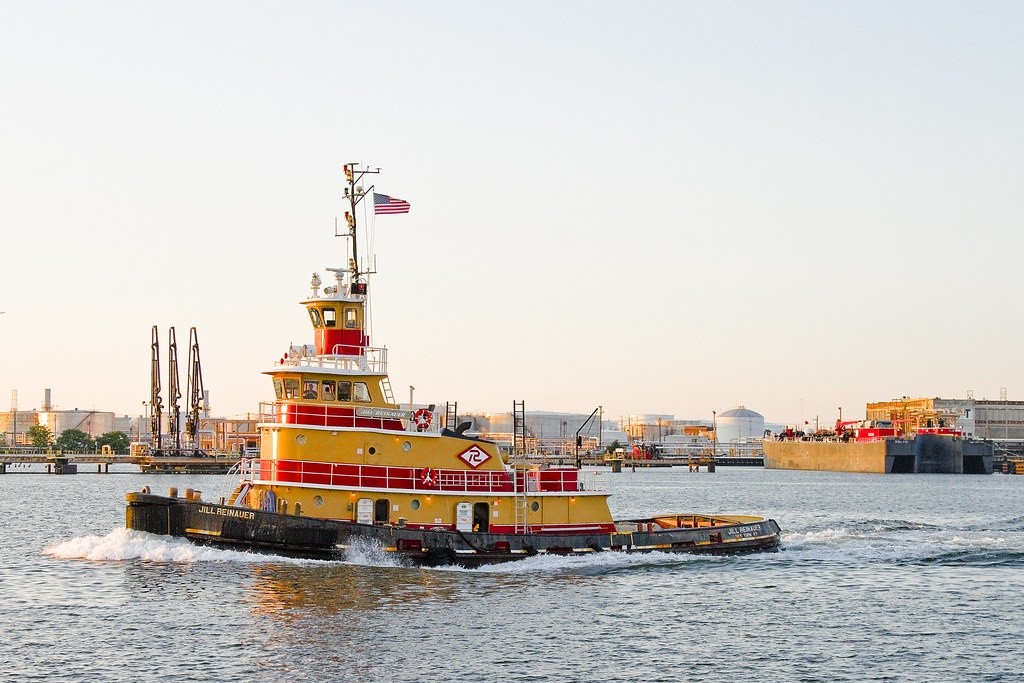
(373, 194), (410, 215)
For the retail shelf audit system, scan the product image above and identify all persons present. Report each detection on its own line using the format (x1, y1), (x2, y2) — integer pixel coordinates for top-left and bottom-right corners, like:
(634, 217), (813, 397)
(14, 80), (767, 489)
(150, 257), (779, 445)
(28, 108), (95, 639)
(323, 387), (336, 402)
(303, 384), (317, 400)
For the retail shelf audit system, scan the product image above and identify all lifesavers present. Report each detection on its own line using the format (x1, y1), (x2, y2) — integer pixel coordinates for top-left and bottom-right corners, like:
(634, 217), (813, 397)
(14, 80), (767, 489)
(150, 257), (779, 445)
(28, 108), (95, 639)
(421, 467), (438, 487)
(414, 409), (431, 428)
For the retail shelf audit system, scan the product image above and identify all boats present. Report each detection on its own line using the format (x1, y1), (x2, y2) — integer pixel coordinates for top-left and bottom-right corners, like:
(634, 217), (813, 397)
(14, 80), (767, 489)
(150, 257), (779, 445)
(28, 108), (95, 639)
(121, 161), (782, 564)
